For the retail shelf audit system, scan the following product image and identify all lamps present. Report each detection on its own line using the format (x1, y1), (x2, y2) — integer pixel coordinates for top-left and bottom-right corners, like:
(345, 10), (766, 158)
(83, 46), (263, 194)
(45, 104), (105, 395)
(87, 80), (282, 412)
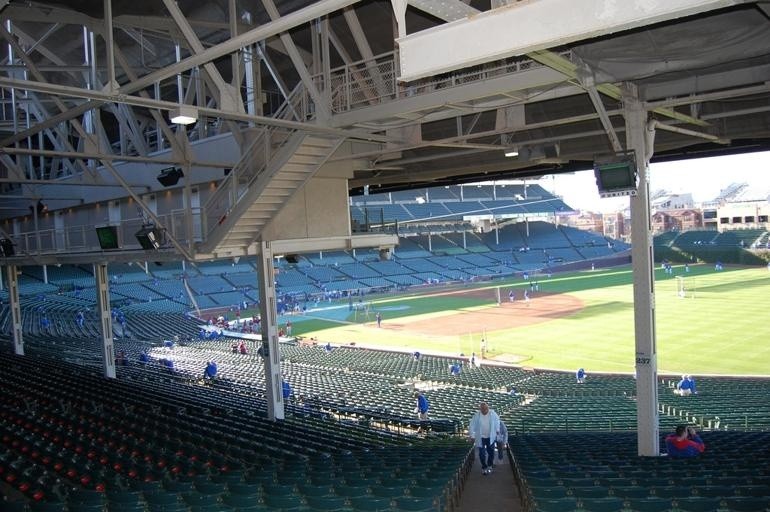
(168, 106), (199, 125)
(503, 145), (519, 157)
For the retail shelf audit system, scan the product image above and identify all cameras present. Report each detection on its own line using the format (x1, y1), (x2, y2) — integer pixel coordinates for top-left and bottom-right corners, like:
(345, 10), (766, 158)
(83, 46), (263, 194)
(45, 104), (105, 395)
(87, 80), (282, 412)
(687, 427), (691, 433)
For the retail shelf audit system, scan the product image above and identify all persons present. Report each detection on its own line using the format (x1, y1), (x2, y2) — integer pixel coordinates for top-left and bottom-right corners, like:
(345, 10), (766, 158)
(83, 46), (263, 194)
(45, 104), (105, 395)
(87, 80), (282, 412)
(201, 361), (216, 379)
(471, 351), (476, 369)
(448, 364), (459, 376)
(210, 360), (216, 367)
(666, 426), (704, 459)
(687, 375), (697, 395)
(481, 338), (486, 359)
(281, 377), (291, 401)
(494, 421), (509, 459)
(468, 402), (501, 476)
(678, 375), (691, 397)
(413, 390), (428, 419)
(577, 367), (584, 384)
(78, 310), (84, 324)
(413, 351), (422, 361)
(35, 271), (192, 369)
(192, 285), (380, 355)
(380, 268), (503, 294)
(509, 272), (540, 302)
(660, 240), (724, 276)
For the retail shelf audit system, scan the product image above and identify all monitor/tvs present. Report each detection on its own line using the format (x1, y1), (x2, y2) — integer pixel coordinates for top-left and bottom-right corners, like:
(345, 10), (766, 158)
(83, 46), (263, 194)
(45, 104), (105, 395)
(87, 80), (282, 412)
(95, 226), (122, 249)
(594, 160), (636, 199)
(135, 229), (161, 250)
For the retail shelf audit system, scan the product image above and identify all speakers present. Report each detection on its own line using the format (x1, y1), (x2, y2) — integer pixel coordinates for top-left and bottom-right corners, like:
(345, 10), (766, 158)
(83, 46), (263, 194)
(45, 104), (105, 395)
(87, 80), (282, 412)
(29, 202), (44, 213)
(223, 169), (233, 176)
(286, 255), (300, 263)
(155, 262), (164, 266)
(157, 168), (179, 186)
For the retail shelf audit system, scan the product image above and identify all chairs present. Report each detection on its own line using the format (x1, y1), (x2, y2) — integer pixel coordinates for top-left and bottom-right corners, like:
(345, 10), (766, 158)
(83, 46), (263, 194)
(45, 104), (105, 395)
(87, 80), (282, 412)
(0, 262), (261, 344)
(274, 184), (633, 299)
(0, 344), (770, 512)
